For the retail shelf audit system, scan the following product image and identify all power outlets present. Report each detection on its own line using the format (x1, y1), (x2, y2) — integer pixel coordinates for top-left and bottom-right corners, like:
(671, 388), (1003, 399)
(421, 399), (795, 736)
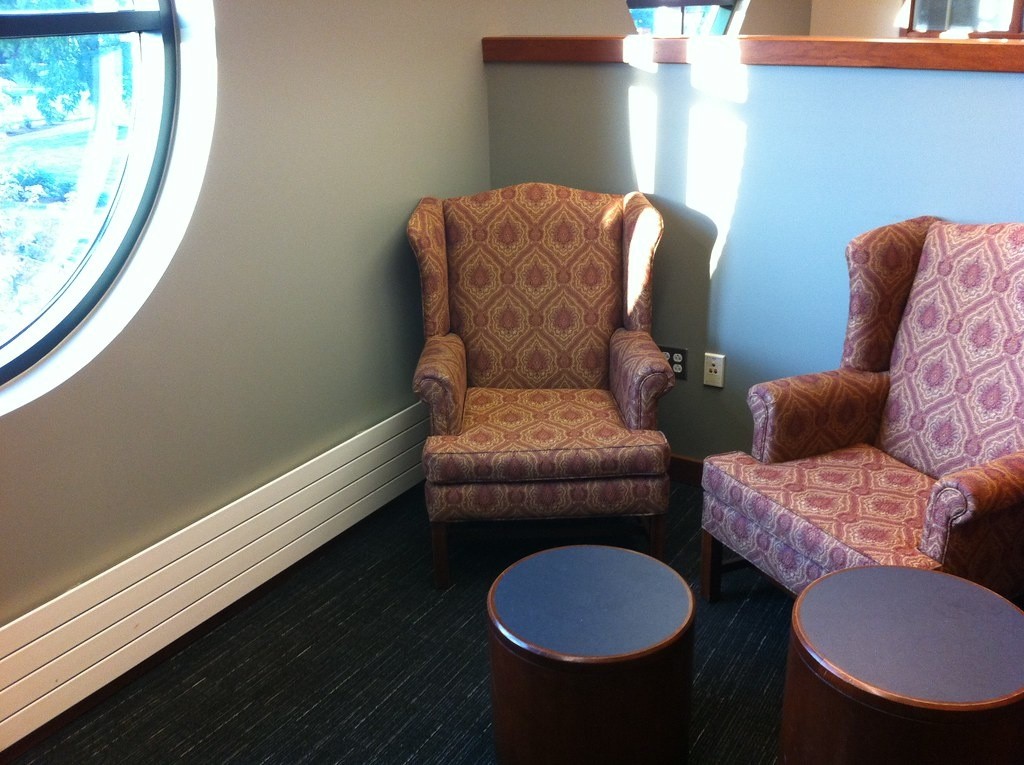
(703, 352), (726, 389)
(658, 344), (688, 382)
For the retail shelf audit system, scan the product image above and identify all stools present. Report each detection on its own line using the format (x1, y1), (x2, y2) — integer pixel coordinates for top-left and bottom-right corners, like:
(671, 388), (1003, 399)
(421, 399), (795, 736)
(481, 541), (698, 765)
(776, 565), (1024, 765)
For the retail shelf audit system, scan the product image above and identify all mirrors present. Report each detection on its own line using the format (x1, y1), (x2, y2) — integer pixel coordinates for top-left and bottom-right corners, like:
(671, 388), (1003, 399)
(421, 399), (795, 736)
(899, 0), (1024, 41)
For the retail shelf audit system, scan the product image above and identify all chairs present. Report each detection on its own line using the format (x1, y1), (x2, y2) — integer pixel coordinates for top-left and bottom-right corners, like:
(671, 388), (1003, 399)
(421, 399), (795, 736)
(695, 214), (1024, 603)
(406, 180), (675, 582)
